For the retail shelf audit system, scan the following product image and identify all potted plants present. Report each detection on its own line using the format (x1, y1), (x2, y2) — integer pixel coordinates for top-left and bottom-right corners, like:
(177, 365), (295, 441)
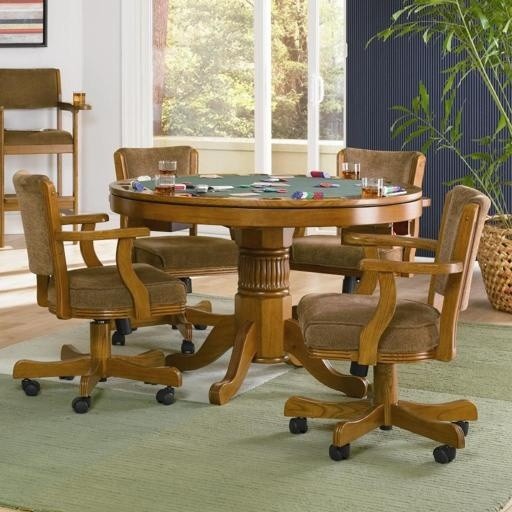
(366, 1), (512, 315)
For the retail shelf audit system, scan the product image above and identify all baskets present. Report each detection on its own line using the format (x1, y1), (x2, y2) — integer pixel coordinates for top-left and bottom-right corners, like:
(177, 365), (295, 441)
(476, 214), (512, 313)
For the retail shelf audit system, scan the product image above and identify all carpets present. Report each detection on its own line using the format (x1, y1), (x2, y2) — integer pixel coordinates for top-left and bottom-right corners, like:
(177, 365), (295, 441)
(2, 291), (512, 512)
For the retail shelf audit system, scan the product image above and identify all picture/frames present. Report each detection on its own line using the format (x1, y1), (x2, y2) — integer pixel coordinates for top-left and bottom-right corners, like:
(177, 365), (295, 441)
(0, 0), (47, 49)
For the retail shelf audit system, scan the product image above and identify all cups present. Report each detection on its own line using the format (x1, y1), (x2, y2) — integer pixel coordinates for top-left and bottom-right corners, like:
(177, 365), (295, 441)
(73, 89), (86, 106)
(361, 176), (384, 200)
(342, 162), (360, 181)
(158, 159), (177, 175)
(154, 174), (175, 198)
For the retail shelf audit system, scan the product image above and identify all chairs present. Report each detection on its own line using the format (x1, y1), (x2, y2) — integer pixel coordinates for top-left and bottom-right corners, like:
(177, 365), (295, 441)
(284, 184), (492, 465)
(112, 143), (243, 354)
(290, 146), (432, 296)
(0, 68), (92, 247)
(12, 170), (187, 414)
